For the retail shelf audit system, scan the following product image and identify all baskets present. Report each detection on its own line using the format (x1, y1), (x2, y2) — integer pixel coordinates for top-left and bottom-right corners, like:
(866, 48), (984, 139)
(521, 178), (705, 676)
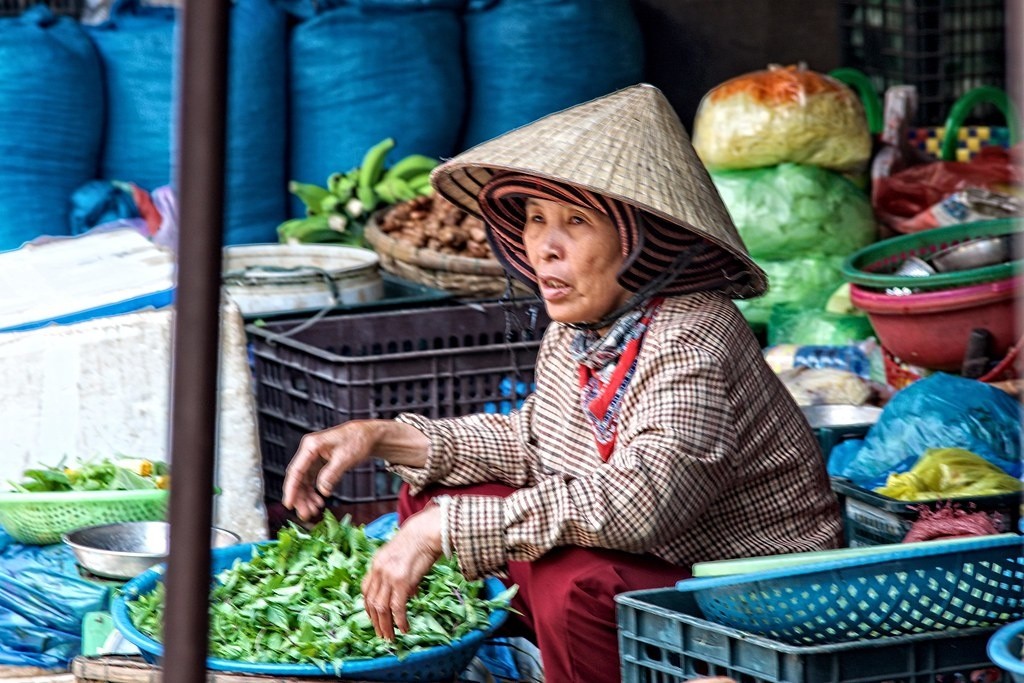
(844, 2), (1005, 122)
(674, 528), (1024, 639)
(839, 216), (1024, 299)
(110, 539), (509, 683)
(0, 485), (164, 542)
(832, 474), (1024, 543)
(366, 206), (530, 297)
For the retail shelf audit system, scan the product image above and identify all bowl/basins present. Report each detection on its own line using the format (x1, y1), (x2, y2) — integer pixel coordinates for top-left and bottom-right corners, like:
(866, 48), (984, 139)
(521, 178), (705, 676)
(850, 239), (1024, 366)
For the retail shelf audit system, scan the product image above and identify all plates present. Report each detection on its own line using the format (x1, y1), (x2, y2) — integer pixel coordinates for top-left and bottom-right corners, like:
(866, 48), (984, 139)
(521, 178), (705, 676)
(801, 405), (884, 431)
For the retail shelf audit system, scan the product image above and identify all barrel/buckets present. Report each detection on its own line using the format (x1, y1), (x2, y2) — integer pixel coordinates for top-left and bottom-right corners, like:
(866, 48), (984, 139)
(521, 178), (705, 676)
(223, 244), (381, 314)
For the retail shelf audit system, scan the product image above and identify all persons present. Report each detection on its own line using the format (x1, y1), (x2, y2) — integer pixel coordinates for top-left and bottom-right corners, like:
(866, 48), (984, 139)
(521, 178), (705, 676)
(283, 83), (850, 683)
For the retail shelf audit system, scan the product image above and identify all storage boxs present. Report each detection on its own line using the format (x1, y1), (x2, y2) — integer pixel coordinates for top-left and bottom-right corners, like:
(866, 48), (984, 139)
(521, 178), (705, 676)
(245, 293), (554, 512)
(615, 588), (1015, 683)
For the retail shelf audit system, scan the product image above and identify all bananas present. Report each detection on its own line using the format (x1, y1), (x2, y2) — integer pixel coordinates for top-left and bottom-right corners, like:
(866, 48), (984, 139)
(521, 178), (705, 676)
(276, 138), (441, 246)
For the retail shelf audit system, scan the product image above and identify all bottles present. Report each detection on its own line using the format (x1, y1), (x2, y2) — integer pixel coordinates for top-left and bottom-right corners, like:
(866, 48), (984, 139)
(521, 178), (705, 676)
(761, 344), (872, 380)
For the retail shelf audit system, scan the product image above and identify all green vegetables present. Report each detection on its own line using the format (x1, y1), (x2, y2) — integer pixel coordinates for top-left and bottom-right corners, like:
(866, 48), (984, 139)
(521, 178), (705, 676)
(128, 508), (524, 673)
(6, 462), (169, 491)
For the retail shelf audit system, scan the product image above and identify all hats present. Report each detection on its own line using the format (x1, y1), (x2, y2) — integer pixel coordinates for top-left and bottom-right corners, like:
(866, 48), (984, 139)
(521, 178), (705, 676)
(432, 84), (769, 299)
